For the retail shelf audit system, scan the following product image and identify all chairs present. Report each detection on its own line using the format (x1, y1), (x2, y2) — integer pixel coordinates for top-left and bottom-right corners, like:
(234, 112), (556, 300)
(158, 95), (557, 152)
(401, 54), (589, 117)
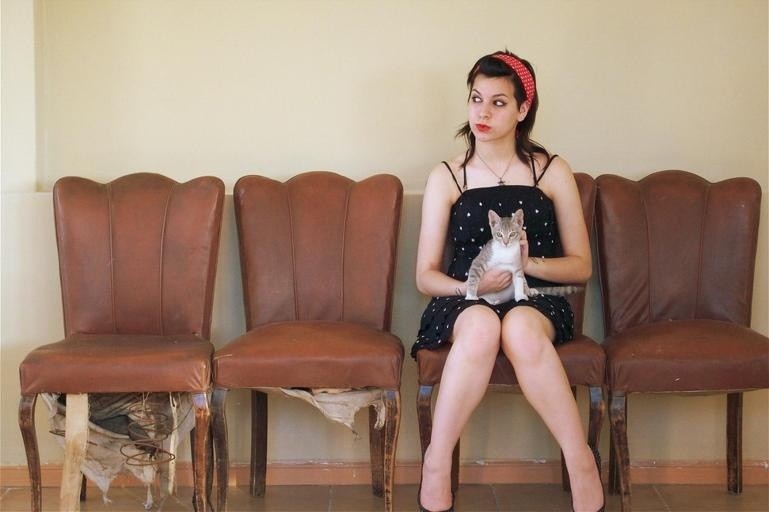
(18, 171), (227, 510)
(596, 170), (769, 496)
(416, 172), (608, 496)
(211, 172), (404, 512)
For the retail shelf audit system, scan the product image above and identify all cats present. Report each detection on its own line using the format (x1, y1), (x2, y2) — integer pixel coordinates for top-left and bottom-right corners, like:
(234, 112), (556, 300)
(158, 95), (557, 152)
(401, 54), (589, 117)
(465, 209), (585, 305)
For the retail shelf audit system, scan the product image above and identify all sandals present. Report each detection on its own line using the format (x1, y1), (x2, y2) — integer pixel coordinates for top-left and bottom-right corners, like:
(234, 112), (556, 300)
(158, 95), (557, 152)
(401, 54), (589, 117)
(417, 444), (455, 512)
(568, 444), (604, 512)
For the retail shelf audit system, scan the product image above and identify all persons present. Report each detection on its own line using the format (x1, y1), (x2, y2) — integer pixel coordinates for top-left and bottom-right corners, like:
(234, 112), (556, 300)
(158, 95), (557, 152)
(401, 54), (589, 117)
(413, 47), (606, 512)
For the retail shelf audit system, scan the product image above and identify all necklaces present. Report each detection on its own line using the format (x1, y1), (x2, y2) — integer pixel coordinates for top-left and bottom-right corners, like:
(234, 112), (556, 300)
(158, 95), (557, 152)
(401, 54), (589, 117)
(470, 144), (519, 188)
